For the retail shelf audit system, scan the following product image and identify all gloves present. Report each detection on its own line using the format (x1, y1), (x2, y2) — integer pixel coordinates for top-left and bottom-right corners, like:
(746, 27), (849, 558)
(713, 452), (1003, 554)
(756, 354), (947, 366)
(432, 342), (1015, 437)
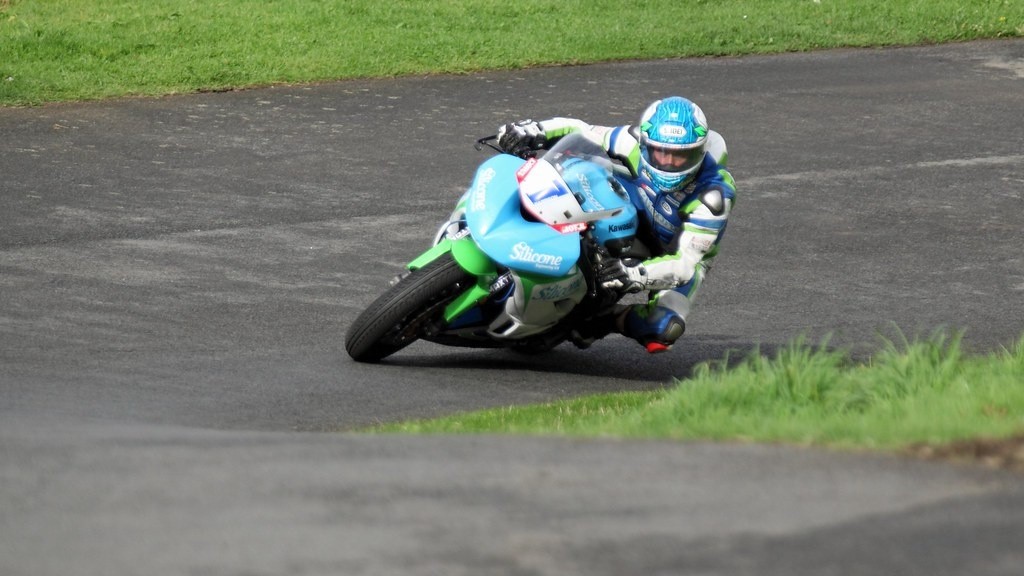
(594, 257), (649, 293)
(497, 118), (548, 155)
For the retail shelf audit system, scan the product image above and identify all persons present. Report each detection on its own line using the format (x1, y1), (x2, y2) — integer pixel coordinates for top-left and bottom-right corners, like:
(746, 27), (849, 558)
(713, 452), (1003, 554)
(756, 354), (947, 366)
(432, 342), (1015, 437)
(443, 96), (736, 353)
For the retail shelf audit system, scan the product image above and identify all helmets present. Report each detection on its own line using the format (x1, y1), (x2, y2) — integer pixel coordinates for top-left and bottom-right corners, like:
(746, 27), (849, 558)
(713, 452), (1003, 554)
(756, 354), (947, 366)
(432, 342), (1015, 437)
(639, 97), (709, 192)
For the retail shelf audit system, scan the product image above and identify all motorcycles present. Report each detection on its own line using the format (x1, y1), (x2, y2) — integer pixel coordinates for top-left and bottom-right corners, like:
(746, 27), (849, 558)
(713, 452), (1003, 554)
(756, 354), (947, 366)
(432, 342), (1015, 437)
(344, 127), (731, 365)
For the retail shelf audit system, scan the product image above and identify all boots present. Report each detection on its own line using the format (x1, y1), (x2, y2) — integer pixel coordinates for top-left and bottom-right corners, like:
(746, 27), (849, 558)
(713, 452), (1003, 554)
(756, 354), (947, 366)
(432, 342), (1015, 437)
(571, 305), (629, 350)
